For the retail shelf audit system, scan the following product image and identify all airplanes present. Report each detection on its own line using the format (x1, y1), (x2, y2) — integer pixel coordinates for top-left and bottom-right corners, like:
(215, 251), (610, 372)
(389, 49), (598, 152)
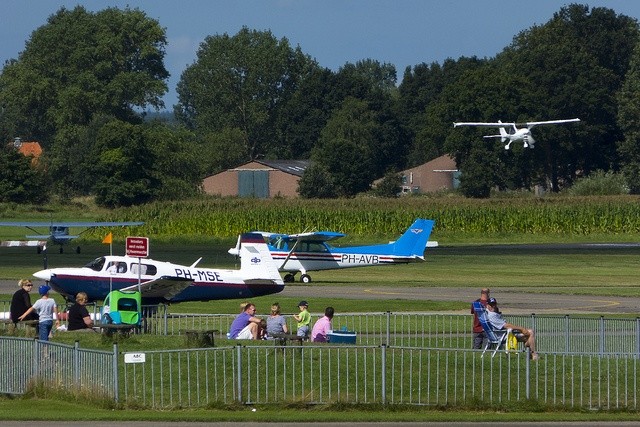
(229, 218), (434, 283)
(0, 221), (144, 244)
(33, 230), (284, 304)
(454, 119), (582, 150)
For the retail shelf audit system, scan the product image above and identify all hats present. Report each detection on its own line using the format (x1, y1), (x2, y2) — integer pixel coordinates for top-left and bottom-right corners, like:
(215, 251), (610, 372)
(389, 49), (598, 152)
(487, 297), (497, 304)
(39, 285), (53, 295)
(296, 301), (309, 307)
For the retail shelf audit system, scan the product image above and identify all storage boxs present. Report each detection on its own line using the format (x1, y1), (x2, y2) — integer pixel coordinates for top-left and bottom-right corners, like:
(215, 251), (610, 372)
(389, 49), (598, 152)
(325, 329), (357, 344)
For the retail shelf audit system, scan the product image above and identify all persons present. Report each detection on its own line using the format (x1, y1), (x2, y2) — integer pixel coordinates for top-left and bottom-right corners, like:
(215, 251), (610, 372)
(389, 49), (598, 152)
(484, 296), (541, 359)
(228, 303), (266, 341)
(293, 300), (311, 338)
(10, 277), (39, 322)
(310, 306), (334, 342)
(266, 301), (288, 346)
(470, 287), (492, 349)
(106, 261), (126, 273)
(17, 284), (60, 342)
(67, 291), (94, 330)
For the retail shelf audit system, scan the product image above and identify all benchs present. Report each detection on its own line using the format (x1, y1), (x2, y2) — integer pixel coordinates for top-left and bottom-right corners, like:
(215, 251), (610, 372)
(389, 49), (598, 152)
(178, 328), (220, 347)
(92, 322), (138, 342)
(0, 318), (40, 337)
(272, 332), (308, 355)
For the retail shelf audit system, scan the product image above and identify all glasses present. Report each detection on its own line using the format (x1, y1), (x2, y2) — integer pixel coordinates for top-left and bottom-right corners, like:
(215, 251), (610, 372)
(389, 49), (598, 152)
(249, 308), (256, 312)
(25, 284), (34, 287)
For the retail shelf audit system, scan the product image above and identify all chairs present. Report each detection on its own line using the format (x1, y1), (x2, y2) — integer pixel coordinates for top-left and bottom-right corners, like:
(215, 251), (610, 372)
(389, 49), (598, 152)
(473, 301), (520, 359)
